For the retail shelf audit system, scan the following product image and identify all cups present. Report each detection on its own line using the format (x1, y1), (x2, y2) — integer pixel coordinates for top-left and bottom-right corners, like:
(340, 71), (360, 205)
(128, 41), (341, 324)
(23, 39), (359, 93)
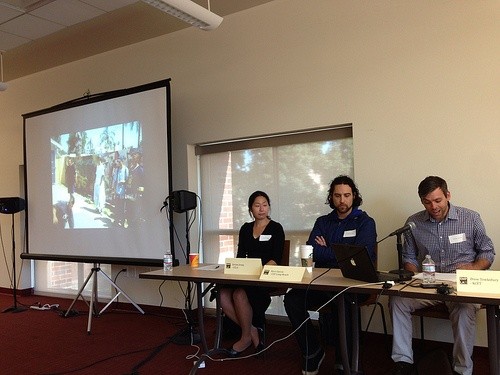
(189, 253), (198, 267)
(300, 244), (314, 273)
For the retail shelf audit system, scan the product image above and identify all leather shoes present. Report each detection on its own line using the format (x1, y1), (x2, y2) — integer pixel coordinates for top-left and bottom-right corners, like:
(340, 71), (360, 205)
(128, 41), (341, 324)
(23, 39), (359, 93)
(252, 327), (264, 360)
(223, 338), (252, 358)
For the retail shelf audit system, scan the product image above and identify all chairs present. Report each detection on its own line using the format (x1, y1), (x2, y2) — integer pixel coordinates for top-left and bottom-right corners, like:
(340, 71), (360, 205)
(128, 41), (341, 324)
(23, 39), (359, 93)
(222, 240), (290, 362)
(359, 292), (388, 352)
(411, 303), (450, 356)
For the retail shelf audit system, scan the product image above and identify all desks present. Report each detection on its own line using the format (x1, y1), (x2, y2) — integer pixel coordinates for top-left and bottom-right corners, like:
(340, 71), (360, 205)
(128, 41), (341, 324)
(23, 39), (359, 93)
(138, 263), (500, 375)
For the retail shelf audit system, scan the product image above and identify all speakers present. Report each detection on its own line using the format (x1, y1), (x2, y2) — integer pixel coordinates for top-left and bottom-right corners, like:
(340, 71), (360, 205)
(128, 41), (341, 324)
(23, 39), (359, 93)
(171, 190), (197, 213)
(320, 305), (364, 347)
(0, 198), (25, 214)
(220, 311), (258, 341)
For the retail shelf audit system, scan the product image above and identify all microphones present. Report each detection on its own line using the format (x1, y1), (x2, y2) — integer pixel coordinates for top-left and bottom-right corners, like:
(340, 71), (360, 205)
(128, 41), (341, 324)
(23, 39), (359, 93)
(390, 221), (416, 236)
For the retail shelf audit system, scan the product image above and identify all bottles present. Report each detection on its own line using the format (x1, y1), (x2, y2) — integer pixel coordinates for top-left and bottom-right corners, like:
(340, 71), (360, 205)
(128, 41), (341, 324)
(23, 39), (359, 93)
(422, 255), (436, 285)
(164, 249), (172, 272)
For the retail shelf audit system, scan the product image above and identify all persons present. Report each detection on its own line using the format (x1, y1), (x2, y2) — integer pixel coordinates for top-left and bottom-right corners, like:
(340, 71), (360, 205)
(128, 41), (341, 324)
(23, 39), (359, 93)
(282, 174), (378, 375)
(388, 175), (495, 375)
(219, 191), (285, 357)
(93, 148), (144, 227)
(65, 158), (77, 205)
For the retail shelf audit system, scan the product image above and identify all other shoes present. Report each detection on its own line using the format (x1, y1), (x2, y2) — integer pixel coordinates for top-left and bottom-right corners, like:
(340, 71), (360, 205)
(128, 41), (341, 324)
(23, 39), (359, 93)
(301, 348), (326, 375)
(388, 361), (418, 375)
(335, 362), (351, 375)
